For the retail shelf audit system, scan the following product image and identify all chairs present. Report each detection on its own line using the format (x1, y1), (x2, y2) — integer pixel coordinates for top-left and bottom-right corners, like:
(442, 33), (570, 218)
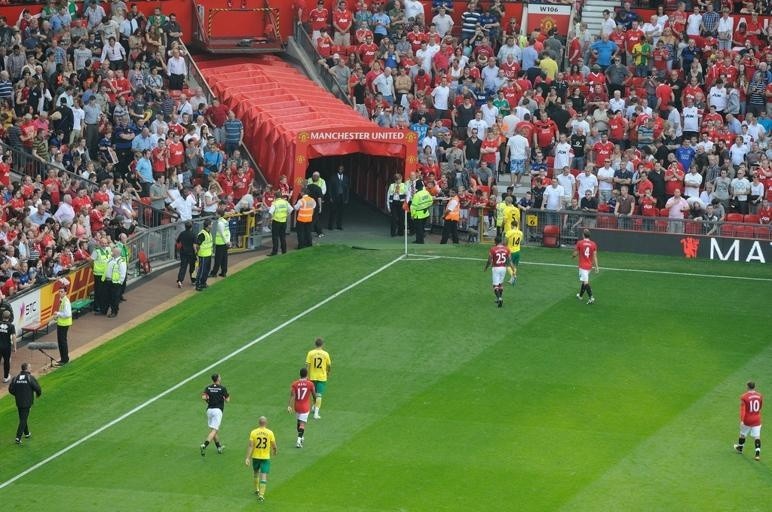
(0, 18), (271, 305)
(302, 12), (770, 246)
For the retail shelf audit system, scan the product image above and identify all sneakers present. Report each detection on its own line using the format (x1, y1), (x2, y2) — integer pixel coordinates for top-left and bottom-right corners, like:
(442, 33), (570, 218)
(267, 253), (274, 257)
(494, 299), (503, 308)
(25, 432), (32, 438)
(257, 496), (264, 503)
(217, 445), (225, 455)
(586, 296), (595, 305)
(734, 443), (743, 454)
(207, 273), (227, 277)
(93, 297), (127, 318)
(15, 438), (24, 444)
(576, 292), (583, 300)
(192, 280), (208, 291)
(200, 444), (207, 457)
(296, 436), (305, 448)
(55, 359), (69, 366)
(506, 279), (517, 285)
(251, 490), (260, 496)
(311, 404), (321, 419)
(751, 455), (760, 461)
(177, 281), (183, 288)
(2, 373), (11, 383)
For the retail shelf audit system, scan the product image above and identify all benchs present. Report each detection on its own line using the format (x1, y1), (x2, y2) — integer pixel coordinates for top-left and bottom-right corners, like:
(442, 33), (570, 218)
(70, 297), (96, 319)
(21, 320), (50, 342)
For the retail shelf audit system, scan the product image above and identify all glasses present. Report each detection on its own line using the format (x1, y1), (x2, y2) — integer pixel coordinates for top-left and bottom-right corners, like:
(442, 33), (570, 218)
(615, 60), (621, 62)
(584, 194), (591, 196)
(603, 161), (611, 163)
(444, 134), (452, 136)
(510, 22), (515, 24)
(737, 172), (743, 175)
(472, 133), (478, 135)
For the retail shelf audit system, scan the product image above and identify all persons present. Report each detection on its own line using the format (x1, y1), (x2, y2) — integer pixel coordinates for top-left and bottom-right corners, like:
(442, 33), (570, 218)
(1, 310), (17, 384)
(244, 416), (278, 502)
(7, 362), (41, 445)
(51, 285), (74, 366)
(287, 368), (317, 449)
(305, 338), (332, 420)
(483, 236), (517, 307)
(0, 290), (14, 364)
(502, 221), (523, 286)
(733, 381), (764, 462)
(570, 228), (599, 306)
(199, 373), (230, 458)
